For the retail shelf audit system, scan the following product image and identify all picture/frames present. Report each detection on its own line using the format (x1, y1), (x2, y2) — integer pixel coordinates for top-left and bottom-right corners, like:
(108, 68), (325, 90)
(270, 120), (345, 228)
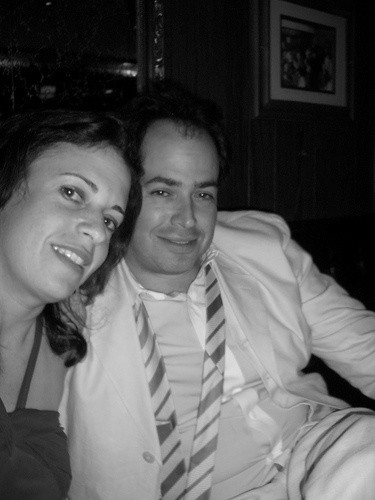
(252, 0), (357, 126)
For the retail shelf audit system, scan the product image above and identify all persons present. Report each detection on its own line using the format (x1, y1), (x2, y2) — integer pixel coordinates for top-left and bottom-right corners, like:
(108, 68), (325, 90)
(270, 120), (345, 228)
(67, 103), (375, 500)
(281, 36), (333, 91)
(0, 113), (142, 500)
